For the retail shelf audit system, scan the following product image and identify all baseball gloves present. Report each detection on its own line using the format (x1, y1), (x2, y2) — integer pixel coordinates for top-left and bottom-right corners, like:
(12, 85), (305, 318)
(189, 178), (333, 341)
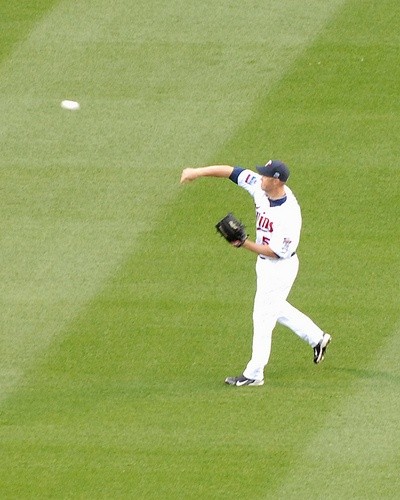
(215, 213), (250, 247)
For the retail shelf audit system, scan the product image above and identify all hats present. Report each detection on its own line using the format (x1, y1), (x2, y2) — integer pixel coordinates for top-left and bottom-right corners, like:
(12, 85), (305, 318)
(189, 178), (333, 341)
(256, 159), (290, 182)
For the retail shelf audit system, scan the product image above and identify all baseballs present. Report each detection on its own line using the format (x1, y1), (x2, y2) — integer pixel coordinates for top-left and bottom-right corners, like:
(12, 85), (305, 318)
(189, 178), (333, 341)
(61, 100), (79, 110)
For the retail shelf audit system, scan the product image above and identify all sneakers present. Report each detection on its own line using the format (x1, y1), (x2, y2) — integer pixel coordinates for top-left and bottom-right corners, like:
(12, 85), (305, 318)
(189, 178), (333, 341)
(313, 332), (333, 364)
(224, 374), (264, 387)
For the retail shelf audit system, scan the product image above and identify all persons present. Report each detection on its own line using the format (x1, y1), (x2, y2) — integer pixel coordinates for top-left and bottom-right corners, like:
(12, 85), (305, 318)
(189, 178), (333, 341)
(179, 159), (332, 387)
